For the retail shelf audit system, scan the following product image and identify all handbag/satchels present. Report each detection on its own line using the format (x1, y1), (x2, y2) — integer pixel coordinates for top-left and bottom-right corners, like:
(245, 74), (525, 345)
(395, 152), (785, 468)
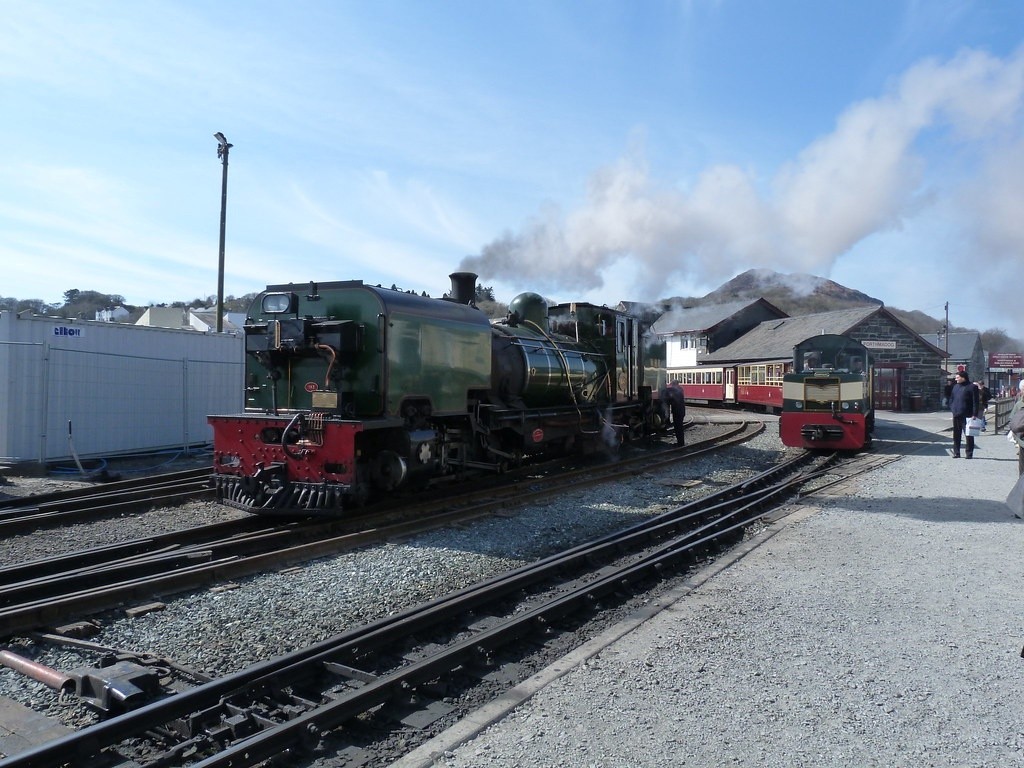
(965, 417), (982, 437)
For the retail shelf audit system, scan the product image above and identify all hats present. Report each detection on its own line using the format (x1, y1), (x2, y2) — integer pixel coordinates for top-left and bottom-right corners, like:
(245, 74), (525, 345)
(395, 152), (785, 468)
(978, 380), (984, 384)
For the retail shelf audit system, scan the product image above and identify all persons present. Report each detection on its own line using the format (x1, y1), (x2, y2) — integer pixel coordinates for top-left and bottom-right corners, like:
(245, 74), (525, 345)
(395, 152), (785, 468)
(999, 385), (1016, 397)
(979, 381), (991, 423)
(668, 379), (685, 446)
(942, 379), (952, 411)
(948, 371), (979, 457)
(973, 382), (985, 431)
(1011, 379), (1024, 519)
(802, 358), (817, 372)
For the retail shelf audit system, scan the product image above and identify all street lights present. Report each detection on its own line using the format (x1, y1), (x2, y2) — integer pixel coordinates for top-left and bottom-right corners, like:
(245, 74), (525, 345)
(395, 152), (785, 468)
(212, 131), (234, 332)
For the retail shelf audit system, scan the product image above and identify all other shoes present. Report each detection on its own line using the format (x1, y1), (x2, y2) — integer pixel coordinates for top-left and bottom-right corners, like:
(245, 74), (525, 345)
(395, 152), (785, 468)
(981, 427), (985, 432)
(965, 454), (972, 459)
(952, 453), (960, 458)
(673, 443), (684, 446)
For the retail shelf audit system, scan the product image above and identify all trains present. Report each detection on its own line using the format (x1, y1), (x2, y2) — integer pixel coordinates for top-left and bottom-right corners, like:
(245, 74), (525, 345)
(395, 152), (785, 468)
(665, 334), (875, 451)
(204, 271), (670, 519)
